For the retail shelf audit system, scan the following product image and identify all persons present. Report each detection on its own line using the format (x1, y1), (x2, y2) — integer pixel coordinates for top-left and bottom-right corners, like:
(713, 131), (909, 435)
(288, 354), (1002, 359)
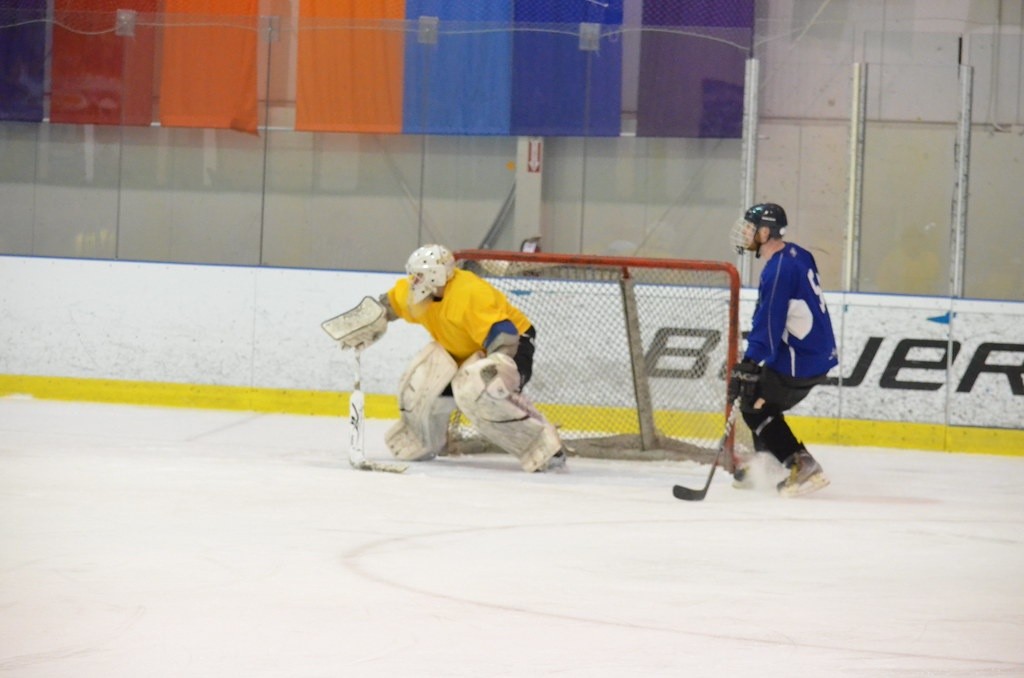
(726, 203), (839, 498)
(322, 244), (566, 473)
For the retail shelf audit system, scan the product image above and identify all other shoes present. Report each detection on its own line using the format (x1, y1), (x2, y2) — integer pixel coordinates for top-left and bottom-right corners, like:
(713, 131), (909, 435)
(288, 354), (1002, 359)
(539, 453), (566, 471)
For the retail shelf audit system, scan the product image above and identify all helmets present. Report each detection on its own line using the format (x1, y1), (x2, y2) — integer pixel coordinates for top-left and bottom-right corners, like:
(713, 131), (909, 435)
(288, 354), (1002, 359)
(730, 202), (786, 258)
(405, 244), (456, 306)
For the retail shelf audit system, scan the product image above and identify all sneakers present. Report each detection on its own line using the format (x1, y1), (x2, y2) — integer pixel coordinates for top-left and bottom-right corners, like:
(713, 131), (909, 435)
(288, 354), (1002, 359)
(777, 442), (822, 489)
(736, 453), (783, 480)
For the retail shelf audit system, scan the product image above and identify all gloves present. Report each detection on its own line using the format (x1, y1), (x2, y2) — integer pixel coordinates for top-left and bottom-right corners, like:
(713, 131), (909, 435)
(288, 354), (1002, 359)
(461, 350), (522, 400)
(321, 295), (388, 352)
(727, 361), (761, 401)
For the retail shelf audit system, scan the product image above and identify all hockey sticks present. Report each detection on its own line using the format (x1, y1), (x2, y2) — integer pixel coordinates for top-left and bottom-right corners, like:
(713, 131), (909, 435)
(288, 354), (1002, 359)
(346, 345), (411, 475)
(671, 391), (743, 503)
